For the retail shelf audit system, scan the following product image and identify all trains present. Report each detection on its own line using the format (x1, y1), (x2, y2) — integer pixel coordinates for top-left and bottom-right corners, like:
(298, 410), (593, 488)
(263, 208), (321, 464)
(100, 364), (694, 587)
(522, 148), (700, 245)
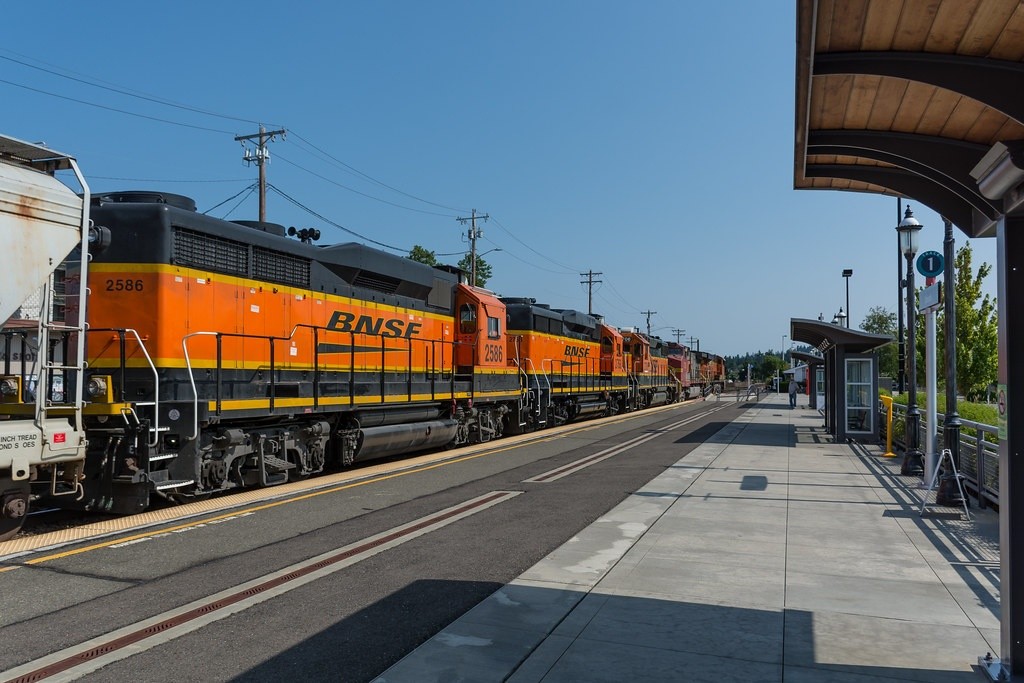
(0, 133), (727, 541)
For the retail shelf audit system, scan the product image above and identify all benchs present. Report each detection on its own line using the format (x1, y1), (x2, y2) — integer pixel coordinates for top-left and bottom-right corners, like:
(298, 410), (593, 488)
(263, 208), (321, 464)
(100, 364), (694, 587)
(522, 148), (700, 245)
(848, 411), (867, 429)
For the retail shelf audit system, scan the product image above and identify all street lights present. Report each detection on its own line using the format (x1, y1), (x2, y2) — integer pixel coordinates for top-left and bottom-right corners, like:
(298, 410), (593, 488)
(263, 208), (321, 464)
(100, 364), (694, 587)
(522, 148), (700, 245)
(842, 268), (853, 329)
(469, 248), (502, 288)
(781, 335), (787, 361)
(893, 203), (925, 477)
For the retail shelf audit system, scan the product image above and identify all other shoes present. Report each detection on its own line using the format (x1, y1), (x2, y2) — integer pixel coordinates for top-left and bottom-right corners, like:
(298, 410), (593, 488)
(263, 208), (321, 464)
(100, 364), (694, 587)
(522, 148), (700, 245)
(791, 406), (797, 409)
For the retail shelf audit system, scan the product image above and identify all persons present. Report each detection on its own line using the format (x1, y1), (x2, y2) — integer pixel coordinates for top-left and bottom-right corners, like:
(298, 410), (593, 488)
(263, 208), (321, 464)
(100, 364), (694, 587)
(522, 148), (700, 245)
(789, 378), (799, 407)
